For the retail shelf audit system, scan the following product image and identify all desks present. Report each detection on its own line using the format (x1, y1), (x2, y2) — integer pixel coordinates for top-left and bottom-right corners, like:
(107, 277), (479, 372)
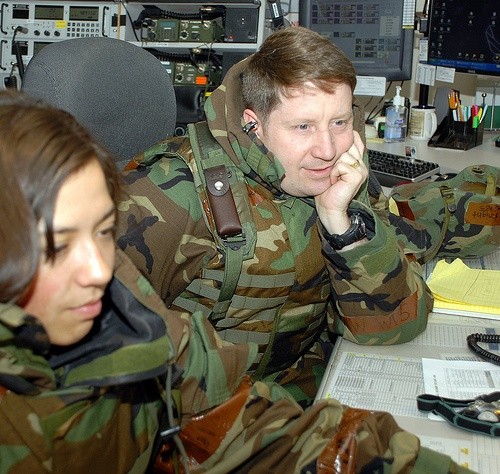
(315, 246), (500, 474)
(367, 130), (500, 175)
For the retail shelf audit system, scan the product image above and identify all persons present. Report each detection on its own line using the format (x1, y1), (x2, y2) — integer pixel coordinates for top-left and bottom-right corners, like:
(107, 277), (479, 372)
(114, 29), (435, 383)
(0, 95), (480, 474)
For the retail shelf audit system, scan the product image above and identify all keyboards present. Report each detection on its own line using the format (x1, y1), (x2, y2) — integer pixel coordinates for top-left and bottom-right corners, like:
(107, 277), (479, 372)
(367, 149), (440, 183)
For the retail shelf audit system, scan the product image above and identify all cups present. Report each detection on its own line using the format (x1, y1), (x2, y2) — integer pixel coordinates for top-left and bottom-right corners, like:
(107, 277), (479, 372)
(409, 105), (437, 140)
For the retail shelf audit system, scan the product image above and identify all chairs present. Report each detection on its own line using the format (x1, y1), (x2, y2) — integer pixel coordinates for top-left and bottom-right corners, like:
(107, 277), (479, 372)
(21, 37), (177, 162)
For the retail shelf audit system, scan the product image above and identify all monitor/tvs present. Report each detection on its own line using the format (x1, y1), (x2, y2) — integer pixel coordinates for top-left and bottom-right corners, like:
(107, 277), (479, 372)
(428, 0), (500, 76)
(298, 0), (414, 82)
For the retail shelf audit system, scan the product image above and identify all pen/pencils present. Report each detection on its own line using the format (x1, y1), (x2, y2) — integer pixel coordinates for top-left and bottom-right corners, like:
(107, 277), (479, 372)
(447, 89), (482, 128)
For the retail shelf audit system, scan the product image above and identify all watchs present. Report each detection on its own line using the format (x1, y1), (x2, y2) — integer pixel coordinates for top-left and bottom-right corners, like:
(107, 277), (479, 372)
(318, 214), (369, 246)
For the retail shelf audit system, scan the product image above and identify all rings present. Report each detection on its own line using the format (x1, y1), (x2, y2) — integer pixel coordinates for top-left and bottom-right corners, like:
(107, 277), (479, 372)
(350, 160), (361, 167)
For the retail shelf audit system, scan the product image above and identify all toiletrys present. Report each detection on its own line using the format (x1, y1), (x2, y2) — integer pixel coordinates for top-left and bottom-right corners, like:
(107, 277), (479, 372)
(384, 85), (408, 143)
